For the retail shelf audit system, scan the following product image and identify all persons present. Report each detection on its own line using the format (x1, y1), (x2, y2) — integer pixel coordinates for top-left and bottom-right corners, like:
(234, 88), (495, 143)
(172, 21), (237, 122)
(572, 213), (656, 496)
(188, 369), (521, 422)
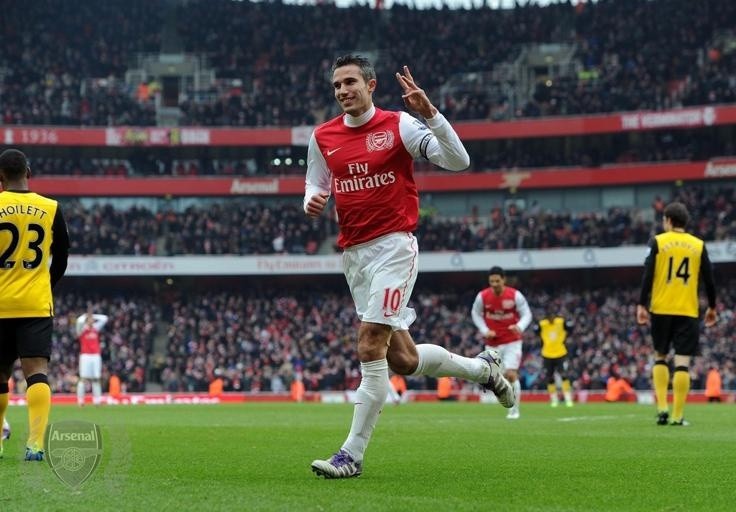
(1, 0), (735, 478)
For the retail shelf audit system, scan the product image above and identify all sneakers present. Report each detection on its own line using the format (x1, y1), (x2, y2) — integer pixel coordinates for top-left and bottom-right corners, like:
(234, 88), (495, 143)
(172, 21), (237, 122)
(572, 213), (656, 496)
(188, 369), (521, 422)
(657, 410), (690, 427)
(479, 349), (516, 408)
(1, 420), (10, 457)
(25, 447), (44, 460)
(311, 451), (363, 479)
